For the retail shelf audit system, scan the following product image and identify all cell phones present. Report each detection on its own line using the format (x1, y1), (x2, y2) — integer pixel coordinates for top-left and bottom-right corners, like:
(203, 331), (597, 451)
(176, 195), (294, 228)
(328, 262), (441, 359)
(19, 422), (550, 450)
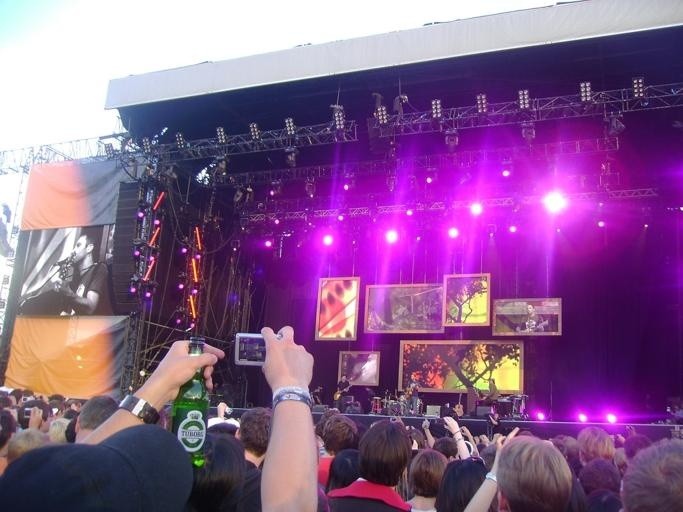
(24, 408), (31, 419)
(22, 397), (27, 402)
(435, 418), (446, 424)
(234, 332), (282, 367)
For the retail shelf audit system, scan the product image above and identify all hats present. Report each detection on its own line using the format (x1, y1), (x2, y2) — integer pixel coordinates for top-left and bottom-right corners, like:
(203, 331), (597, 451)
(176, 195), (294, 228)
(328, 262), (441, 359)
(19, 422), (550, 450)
(0, 424), (194, 511)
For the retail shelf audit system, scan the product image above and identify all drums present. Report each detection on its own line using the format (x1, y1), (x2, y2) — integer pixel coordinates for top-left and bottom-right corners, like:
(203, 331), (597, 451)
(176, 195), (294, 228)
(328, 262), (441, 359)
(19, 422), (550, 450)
(389, 402), (402, 414)
(400, 395), (406, 401)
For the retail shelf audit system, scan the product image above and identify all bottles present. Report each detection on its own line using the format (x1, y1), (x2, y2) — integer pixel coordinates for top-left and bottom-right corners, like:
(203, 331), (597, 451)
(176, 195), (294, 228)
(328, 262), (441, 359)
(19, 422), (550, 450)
(170, 335), (209, 470)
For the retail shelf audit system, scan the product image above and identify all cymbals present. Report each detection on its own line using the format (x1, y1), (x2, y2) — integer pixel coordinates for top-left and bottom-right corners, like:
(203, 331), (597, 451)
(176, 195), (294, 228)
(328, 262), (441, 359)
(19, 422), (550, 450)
(372, 396), (381, 400)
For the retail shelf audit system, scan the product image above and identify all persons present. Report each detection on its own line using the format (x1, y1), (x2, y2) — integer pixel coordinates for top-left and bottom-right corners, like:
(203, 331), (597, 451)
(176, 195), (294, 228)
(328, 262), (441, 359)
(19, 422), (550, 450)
(516, 303), (548, 332)
(54, 231), (113, 315)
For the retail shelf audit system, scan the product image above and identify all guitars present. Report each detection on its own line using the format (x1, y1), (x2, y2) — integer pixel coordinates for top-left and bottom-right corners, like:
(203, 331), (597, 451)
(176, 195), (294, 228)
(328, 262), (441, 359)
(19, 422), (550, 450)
(454, 393), (463, 417)
(333, 384), (352, 400)
(519, 320), (548, 332)
(57, 257), (74, 316)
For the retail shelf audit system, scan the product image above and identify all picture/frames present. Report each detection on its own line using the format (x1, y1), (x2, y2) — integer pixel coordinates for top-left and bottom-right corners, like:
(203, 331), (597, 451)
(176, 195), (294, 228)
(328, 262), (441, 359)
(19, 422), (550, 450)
(363, 273), (562, 338)
(396, 338), (525, 396)
(335, 350), (380, 388)
(313, 276), (361, 341)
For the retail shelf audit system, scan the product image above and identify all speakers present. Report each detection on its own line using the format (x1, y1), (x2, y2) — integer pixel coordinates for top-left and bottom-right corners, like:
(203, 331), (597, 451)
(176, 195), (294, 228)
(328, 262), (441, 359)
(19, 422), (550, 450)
(427, 405), (442, 418)
(311, 404), (328, 412)
(476, 400), (494, 416)
